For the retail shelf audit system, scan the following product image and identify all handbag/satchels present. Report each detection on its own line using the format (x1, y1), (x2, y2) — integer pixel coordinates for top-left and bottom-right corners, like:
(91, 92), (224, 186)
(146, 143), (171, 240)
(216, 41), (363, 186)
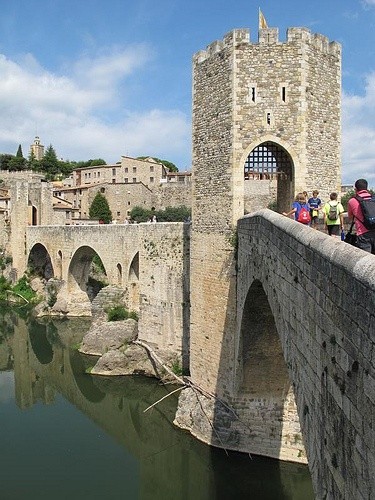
(344, 232), (357, 245)
(312, 210), (318, 217)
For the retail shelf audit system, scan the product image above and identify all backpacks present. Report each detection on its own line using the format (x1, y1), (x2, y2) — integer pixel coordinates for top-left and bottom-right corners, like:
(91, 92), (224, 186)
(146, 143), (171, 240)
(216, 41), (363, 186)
(327, 202), (340, 220)
(352, 194), (375, 230)
(298, 202), (311, 223)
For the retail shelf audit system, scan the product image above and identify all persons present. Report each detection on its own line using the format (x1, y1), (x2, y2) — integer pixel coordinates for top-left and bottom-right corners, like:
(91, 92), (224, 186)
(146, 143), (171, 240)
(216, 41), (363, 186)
(124, 218), (129, 224)
(282, 191), (310, 223)
(99, 217), (109, 224)
(348, 179), (375, 255)
(323, 192), (345, 236)
(308, 190), (322, 230)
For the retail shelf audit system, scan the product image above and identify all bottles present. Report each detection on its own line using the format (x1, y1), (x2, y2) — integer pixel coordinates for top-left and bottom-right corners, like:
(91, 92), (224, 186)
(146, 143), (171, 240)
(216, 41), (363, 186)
(341, 230), (344, 241)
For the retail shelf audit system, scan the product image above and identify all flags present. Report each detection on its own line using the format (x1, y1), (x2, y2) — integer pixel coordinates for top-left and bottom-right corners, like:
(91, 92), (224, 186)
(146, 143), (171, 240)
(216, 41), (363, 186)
(259, 11), (267, 28)
